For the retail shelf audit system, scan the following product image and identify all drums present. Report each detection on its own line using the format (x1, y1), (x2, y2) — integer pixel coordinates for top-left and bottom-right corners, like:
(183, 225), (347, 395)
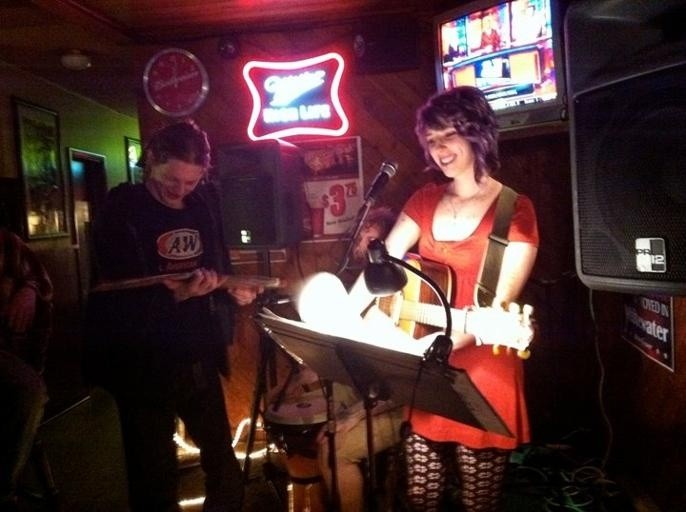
(263, 391), (347, 511)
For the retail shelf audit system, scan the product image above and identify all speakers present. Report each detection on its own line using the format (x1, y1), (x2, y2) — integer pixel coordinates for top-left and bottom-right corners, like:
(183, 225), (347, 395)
(570, 62), (686, 296)
(211, 138), (304, 250)
(352, 13), (421, 73)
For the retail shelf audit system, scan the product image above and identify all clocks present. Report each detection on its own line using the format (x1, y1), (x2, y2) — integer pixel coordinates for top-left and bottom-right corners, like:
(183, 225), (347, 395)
(140, 48), (211, 119)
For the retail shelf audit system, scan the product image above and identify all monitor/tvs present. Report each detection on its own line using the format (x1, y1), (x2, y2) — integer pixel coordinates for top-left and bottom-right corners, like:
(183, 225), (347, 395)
(432, 0), (568, 130)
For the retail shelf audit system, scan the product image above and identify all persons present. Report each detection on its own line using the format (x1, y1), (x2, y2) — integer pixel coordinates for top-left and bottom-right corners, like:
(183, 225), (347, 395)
(349, 87), (539, 512)
(0, 233), (53, 356)
(88, 118), (264, 512)
(318, 210), (422, 512)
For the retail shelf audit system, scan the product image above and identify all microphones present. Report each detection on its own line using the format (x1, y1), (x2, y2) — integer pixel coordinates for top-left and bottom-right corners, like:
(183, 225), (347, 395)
(364, 158), (399, 207)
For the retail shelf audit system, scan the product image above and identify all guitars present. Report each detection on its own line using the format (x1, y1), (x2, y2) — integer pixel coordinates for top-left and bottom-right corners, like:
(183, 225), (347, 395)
(374, 251), (540, 360)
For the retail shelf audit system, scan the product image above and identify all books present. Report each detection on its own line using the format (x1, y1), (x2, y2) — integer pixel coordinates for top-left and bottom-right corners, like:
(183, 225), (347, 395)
(93, 269), (281, 294)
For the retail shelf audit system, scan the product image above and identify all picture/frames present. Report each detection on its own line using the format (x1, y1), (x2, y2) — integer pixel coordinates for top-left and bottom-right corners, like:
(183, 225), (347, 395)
(10, 93), (73, 242)
(124, 136), (146, 185)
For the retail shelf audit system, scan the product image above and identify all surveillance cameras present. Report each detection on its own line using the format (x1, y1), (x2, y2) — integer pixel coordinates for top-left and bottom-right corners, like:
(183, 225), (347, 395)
(215, 36), (239, 61)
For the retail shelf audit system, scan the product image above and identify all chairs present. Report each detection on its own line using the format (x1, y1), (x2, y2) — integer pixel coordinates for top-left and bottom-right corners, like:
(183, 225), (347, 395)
(0, 350), (50, 505)
